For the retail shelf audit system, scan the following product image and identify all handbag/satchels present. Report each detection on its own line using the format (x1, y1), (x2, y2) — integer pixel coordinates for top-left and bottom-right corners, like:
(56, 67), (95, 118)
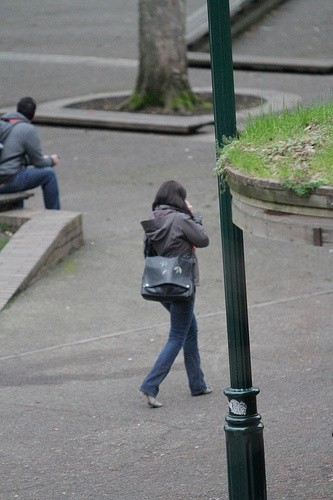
(141, 256), (194, 300)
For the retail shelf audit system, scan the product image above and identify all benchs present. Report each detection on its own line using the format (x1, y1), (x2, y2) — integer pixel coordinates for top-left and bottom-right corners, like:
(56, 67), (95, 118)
(0, 191), (34, 211)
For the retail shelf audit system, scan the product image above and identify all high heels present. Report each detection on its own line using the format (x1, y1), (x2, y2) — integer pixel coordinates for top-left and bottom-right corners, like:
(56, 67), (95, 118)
(139, 390), (162, 407)
(204, 389), (212, 394)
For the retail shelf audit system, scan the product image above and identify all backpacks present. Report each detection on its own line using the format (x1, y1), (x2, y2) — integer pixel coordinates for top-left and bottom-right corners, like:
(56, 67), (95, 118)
(0, 119), (26, 165)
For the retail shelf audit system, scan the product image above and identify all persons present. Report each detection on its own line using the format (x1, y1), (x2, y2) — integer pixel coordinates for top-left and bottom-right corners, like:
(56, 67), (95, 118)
(138, 180), (213, 408)
(0, 96), (63, 210)
